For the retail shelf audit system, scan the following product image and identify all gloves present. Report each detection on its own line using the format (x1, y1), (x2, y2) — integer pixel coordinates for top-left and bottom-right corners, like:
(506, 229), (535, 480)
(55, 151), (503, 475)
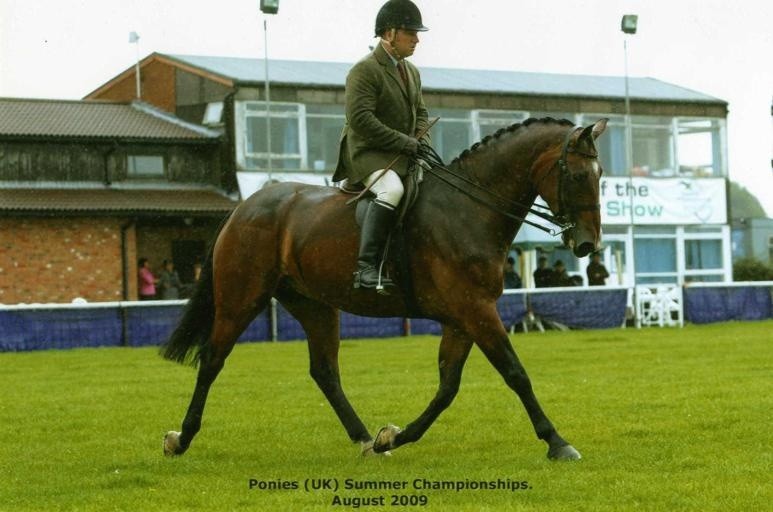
(418, 139), (430, 157)
(403, 136), (420, 158)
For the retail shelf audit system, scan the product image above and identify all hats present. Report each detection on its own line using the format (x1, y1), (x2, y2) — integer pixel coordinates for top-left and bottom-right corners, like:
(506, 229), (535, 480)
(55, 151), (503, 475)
(373, 0), (428, 36)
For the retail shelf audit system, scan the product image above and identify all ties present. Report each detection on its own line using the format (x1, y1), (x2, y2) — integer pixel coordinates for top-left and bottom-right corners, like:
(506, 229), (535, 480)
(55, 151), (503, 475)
(398, 62), (407, 85)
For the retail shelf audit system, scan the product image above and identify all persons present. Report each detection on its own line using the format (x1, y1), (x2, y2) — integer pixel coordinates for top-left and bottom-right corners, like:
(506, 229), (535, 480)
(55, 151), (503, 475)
(533, 256), (553, 285)
(505, 257), (522, 287)
(333, 0), (430, 287)
(158, 258), (183, 299)
(136, 257), (159, 299)
(586, 251), (609, 285)
(554, 259), (568, 285)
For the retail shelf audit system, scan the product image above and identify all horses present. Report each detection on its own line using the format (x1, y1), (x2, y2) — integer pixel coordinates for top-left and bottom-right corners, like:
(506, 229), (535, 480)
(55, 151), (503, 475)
(162, 115), (610, 464)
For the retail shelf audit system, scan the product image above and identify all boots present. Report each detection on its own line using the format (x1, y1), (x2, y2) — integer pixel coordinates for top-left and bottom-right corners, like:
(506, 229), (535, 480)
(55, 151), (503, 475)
(354, 199), (399, 290)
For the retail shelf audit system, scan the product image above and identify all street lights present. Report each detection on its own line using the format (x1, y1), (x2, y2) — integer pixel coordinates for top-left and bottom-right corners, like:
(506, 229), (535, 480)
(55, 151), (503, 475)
(260, 0), (283, 184)
(618, 11), (640, 286)
